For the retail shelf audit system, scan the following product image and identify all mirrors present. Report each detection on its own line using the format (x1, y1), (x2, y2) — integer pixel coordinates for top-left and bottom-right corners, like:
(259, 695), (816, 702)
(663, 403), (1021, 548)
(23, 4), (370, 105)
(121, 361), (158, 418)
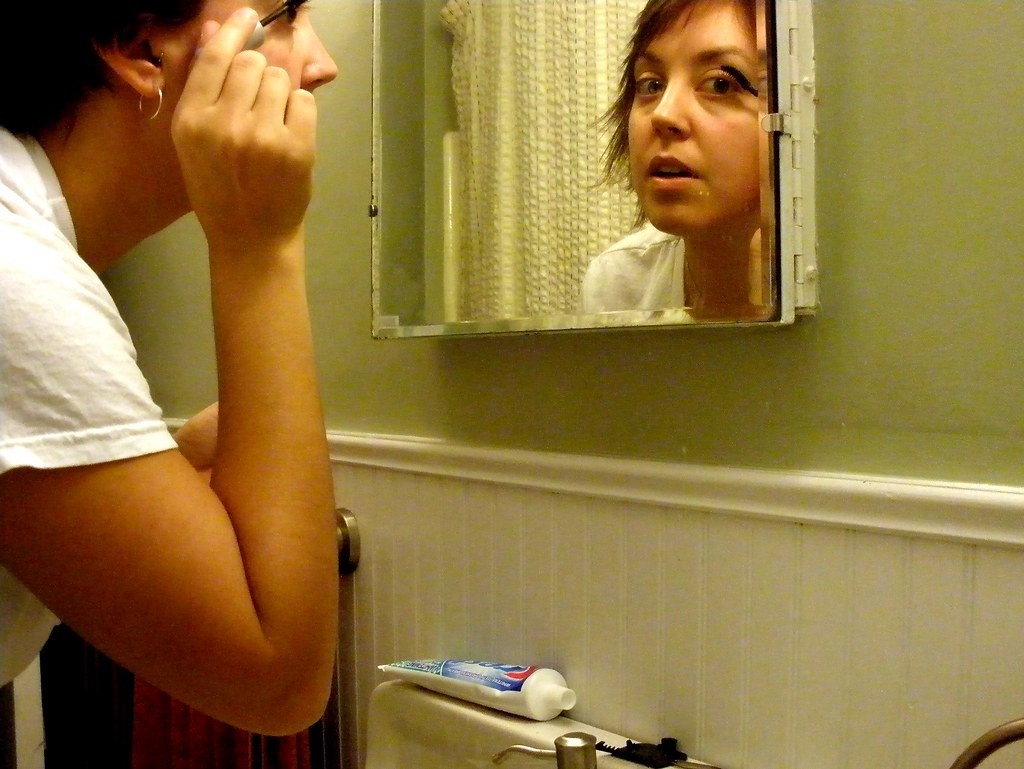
(370, 0), (818, 342)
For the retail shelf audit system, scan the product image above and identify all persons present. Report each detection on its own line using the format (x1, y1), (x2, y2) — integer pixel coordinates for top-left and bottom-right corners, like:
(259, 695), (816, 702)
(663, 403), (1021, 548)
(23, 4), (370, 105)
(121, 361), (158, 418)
(583, 0), (762, 315)
(0, 0), (341, 738)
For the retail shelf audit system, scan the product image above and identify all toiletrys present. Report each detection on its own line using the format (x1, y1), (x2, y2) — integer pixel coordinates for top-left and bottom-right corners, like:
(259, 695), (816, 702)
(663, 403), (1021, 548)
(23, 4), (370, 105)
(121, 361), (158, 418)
(378, 659), (577, 720)
